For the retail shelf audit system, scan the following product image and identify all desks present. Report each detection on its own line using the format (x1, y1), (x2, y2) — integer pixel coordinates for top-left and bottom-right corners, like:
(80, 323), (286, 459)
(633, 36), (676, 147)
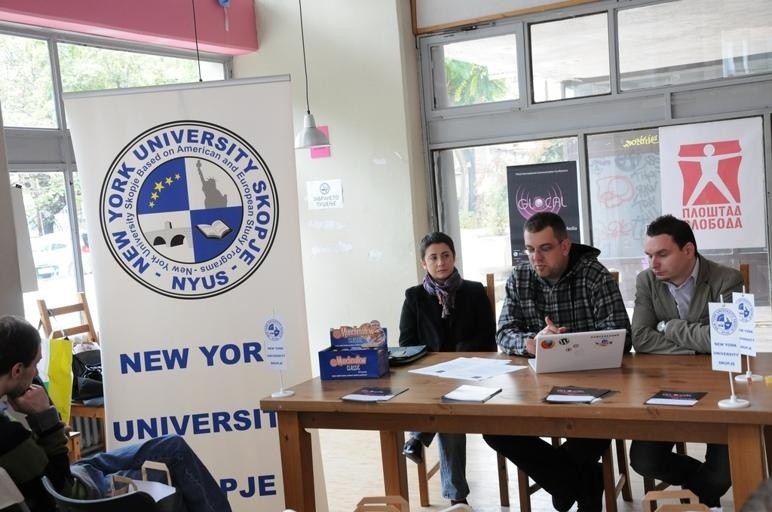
(259, 344), (771, 512)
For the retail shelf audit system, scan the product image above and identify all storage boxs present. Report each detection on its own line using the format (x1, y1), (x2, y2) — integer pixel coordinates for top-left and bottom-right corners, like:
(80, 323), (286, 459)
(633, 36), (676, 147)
(317, 319), (390, 382)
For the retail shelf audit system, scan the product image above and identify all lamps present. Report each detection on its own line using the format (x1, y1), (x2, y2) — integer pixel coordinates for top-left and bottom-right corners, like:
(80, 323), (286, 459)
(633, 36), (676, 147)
(290, 0), (332, 151)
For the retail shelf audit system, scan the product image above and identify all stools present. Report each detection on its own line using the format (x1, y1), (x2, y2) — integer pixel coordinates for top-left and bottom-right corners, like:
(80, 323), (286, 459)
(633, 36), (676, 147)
(62, 429), (81, 462)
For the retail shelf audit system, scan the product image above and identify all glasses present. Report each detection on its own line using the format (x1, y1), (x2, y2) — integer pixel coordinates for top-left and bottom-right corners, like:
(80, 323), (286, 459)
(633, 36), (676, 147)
(524, 240), (563, 256)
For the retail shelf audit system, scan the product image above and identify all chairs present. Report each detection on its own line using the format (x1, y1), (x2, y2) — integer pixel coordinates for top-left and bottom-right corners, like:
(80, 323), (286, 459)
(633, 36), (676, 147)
(646, 263), (754, 512)
(517, 270), (634, 512)
(35, 289), (105, 460)
(411, 273), (514, 508)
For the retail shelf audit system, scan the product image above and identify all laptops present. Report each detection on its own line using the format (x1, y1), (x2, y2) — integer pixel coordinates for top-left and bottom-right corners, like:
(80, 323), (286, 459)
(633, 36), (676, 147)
(527, 328), (627, 374)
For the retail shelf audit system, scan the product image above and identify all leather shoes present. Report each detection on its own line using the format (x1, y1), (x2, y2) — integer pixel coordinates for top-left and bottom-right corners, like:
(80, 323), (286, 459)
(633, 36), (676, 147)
(401, 436), (423, 465)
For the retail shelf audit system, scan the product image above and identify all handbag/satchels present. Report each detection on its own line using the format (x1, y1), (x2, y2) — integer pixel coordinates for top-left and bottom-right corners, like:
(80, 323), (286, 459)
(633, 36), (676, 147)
(74, 363), (103, 401)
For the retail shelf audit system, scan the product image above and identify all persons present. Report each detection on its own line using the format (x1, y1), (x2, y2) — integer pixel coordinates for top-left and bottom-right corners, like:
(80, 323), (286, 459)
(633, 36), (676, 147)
(630, 214), (745, 512)
(399, 232), (495, 508)
(0, 312), (232, 510)
(483, 212), (630, 512)
